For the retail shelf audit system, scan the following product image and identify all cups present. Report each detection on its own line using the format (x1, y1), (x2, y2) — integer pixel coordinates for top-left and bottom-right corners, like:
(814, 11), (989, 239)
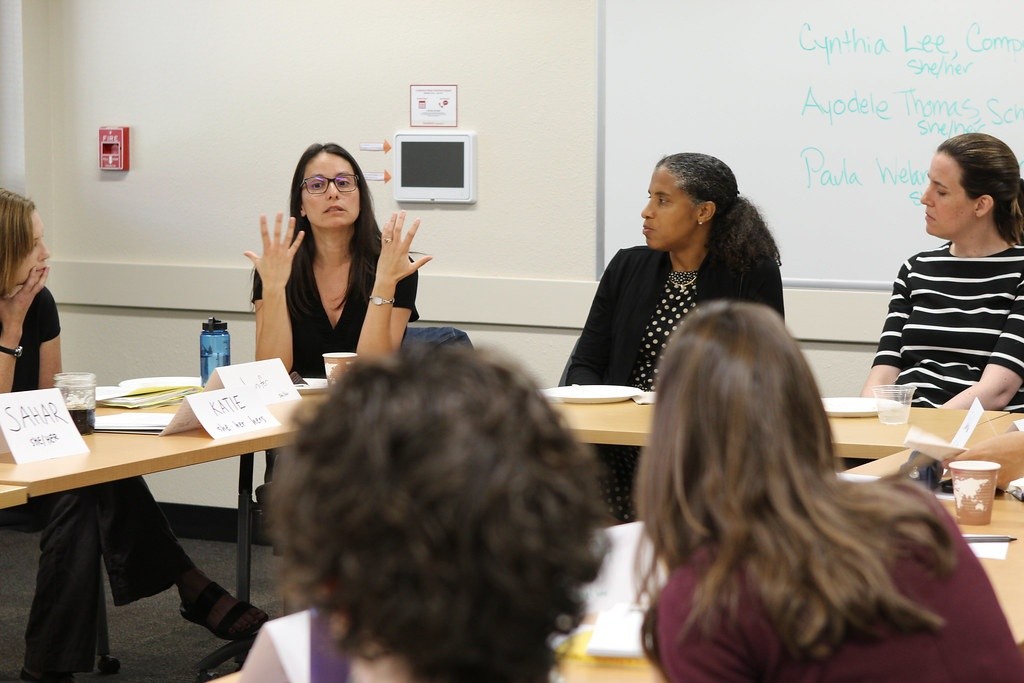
(948, 460), (1002, 526)
(322, 352), (358, 393)
(873, 384), (917, 425)
(52, 372), (97, 436)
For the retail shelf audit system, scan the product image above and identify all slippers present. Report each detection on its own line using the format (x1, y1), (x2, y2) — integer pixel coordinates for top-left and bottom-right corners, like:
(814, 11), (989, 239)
(179, 582), (269, 639)
(20, 665), (74, 683)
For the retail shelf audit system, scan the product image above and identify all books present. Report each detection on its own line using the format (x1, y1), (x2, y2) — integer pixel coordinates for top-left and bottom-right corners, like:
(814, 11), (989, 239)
(101, 386), (203, 408)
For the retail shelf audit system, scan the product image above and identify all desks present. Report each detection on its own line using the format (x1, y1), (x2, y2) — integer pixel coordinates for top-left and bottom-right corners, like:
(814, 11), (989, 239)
(0, 384), (1024, 683)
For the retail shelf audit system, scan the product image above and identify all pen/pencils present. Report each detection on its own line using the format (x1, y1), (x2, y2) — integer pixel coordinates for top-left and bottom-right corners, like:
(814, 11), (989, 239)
(963, 536), (1018, 542)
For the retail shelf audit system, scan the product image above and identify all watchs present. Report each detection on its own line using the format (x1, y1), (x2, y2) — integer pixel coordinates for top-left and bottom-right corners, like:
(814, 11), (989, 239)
(0, 345), (23, 357)
(369, 296), (395, 306)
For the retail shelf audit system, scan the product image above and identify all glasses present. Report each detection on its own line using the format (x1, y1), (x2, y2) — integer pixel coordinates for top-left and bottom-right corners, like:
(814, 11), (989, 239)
(299, 173), (361, 194)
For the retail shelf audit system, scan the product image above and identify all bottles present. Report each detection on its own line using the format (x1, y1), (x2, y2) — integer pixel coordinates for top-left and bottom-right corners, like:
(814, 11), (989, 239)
(200, 317), (231, 388)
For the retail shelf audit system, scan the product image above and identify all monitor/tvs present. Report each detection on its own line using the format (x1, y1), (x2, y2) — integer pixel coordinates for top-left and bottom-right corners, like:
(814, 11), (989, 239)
(392, 133), (477, 205)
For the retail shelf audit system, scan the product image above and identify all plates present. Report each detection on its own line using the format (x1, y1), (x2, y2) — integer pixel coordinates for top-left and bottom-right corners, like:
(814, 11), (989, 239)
(541, 384), (643, 404)
(293, 378), (328, 395)
(118, 376), (200, 390)
(95, 386), (127, 401)
(819, 397), (905, 418)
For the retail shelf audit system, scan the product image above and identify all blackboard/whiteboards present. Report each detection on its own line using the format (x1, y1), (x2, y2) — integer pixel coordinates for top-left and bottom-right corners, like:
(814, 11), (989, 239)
(597, 0), (1023, 292)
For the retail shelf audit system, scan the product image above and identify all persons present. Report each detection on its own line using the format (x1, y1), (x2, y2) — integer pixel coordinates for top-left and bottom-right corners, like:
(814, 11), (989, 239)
(268, 343), (600, 683)
(634, 304), (1024, 683)
(845, 134), (1024, 468)
(940, 431), (1024, 483)
(566, 153), (786, 521)
(244, 143), (432, 510)
(0, 188), (269, 683)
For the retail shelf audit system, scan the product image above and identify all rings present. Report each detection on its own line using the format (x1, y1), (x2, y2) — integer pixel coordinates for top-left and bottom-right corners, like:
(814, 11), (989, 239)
(384, 239), (392, 243)
